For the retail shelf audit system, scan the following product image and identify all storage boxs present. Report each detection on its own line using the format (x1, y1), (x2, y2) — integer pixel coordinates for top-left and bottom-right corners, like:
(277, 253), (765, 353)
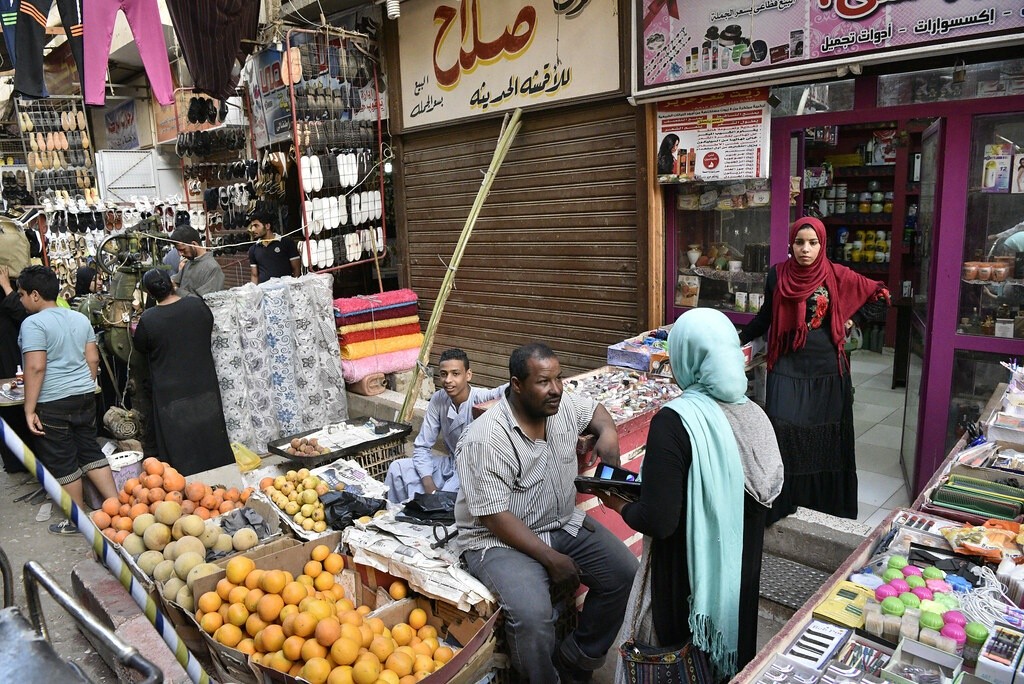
(727, 383), (1024, 684)
(87, 437), (504, 684)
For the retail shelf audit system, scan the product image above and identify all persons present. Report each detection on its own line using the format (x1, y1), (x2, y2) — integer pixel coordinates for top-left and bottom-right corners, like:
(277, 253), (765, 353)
(163, 225), (225, 297)
(66, 266), (117, 439)
(982, 221), (1024, 317)
(454, 343), (640, 684)
(739, 217), (891, 520)
(657, 134), (680, 174)
(592, 307), (788, 684)
(0, 265), (32, 473)
(17, 265), (118, 534)
(1017, 158), (1024, 192)
(248, 211), (300, 285)
(133, 268), (236, 477)
(383, 348), (510, 504)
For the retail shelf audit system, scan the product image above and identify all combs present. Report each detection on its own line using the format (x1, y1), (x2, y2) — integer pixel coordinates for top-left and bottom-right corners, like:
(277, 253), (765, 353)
(936, 472), (1024, 520)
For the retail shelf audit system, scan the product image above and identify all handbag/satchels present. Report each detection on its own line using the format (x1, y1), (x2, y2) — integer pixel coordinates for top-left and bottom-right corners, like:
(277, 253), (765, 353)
(619, 639), (712, 684)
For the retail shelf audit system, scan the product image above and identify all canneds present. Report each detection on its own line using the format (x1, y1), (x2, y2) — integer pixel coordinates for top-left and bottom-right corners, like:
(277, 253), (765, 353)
(813, 184), (848, 213)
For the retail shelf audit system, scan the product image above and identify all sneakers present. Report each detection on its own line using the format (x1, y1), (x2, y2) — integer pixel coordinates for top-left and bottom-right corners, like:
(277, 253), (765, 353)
(48, 518), (81, 534)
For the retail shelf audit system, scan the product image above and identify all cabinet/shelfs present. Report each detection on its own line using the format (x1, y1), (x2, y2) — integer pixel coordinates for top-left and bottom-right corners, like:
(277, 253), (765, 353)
(800, 165), (922, 305)
(673, 206), (774, 281)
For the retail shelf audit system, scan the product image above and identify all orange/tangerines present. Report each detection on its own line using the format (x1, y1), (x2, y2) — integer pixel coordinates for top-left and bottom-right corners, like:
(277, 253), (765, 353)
(197, 545), (454, 684)
(91, 457), (273, 547)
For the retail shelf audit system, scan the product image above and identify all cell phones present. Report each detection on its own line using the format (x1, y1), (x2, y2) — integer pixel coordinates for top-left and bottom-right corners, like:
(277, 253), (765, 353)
(594, 463), (639, 482)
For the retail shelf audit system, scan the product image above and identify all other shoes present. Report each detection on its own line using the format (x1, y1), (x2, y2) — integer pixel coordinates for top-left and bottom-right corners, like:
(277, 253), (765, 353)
(174, 43), (386, 269)
(553, 649), (594, 684)
(0, 111), (174, 293)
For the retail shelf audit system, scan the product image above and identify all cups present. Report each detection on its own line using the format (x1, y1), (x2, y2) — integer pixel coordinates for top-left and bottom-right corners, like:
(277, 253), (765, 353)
(981, 325), (994, 335)
(984, 160), (996, 187)
(728, 260), (742, 272)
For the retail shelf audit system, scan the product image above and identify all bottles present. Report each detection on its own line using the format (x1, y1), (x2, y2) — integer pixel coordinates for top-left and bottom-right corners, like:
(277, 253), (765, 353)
(678, 148), (696, 177)
(15, 365), (25, 388)
(866, 139), (872, 165)
(971, 307), (979, 322)
(2, 380), (17, 391)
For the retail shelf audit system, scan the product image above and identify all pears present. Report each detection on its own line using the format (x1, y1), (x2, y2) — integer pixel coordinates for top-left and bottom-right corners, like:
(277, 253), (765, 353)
(119, 500), (259, 613)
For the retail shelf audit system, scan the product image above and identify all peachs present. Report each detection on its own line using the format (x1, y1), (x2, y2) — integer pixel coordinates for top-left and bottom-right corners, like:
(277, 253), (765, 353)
(286, 438), (330, 457)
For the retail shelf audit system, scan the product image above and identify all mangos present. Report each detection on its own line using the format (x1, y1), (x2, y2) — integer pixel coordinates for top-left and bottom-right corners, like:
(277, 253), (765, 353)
(263, 468), (373, 533)
